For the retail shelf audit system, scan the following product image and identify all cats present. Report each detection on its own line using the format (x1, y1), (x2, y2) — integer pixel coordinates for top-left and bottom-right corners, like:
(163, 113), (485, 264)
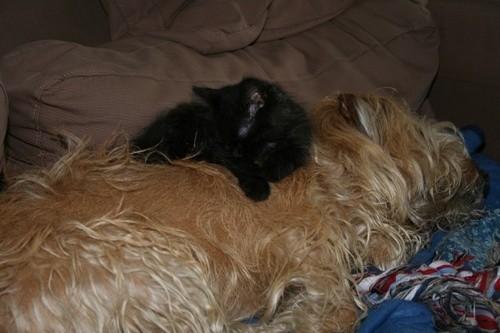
(129, 76), (312, 202)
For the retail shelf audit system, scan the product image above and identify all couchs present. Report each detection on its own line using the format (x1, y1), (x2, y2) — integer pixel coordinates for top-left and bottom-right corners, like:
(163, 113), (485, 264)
(1, 0), (441, 184)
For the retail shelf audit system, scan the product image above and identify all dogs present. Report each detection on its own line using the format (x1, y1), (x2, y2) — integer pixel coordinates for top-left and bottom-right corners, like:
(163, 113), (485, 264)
(0, 86), (489, 333)
